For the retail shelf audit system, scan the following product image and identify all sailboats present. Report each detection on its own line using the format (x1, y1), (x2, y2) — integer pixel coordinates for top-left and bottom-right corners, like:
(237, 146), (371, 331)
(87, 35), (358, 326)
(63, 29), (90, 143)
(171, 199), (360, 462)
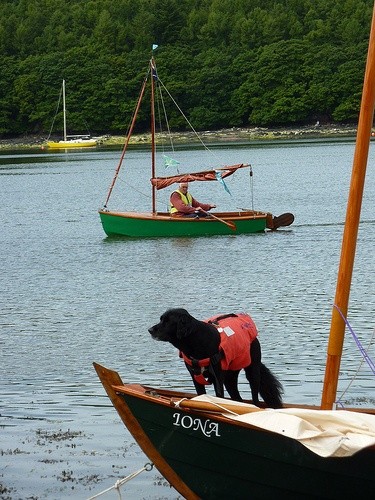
(97, 55), (295, 237)
(93, 6), (375, 499)
(46, 79), (104, 148)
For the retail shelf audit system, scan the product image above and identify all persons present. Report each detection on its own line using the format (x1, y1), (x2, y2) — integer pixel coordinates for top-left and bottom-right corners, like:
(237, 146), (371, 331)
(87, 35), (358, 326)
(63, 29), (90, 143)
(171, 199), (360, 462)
(169, 181), (216, 219)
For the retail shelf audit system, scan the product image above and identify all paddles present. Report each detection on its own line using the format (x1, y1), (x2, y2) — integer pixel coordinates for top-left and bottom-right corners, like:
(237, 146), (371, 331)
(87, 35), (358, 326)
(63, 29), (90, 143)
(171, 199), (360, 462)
(199, 209), (236, 231)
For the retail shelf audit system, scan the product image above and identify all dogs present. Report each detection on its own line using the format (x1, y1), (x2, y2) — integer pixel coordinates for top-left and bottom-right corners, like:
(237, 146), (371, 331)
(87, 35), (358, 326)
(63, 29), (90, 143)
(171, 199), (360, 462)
(148, 308), (286, 409)
